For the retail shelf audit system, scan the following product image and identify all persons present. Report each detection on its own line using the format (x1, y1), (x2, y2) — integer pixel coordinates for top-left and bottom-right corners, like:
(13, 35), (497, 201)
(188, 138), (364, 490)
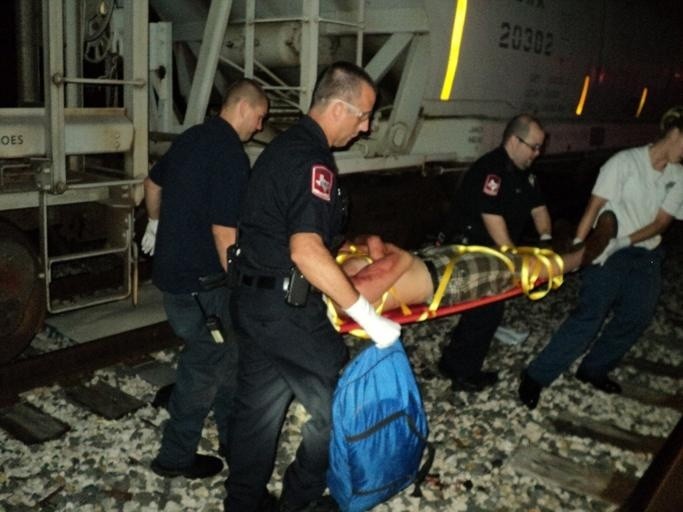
(220, 60), (403, 512)
(432, 110), (547, 396)
(136, 75), (271, 480)
(518, 107), (682, 410)
(333, 211), (615, 322)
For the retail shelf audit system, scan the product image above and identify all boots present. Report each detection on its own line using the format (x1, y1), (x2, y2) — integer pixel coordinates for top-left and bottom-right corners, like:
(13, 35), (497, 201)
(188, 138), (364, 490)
(571, 210), (618, 272)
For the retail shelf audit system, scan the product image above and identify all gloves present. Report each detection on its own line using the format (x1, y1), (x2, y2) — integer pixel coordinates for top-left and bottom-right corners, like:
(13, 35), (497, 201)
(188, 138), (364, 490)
(140, 217), (159, 257)
(591, 234), (632, 266)
(344, 293), (403, 350)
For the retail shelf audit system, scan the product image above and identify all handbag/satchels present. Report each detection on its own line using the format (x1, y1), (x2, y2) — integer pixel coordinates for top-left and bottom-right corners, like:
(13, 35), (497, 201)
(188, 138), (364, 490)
(325, 338), (437, 512)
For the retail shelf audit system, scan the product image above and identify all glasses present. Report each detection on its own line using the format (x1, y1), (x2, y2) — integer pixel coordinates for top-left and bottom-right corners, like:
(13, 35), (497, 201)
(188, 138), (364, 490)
(515, 135), (540, 151)
(335, 97), (371, 121)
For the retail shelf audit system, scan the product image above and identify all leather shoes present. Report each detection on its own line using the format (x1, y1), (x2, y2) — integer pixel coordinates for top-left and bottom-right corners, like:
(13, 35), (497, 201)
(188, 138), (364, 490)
(574, 364), (623, 396)
(517, 369), (544, 410)
(436, 353), (499, 393)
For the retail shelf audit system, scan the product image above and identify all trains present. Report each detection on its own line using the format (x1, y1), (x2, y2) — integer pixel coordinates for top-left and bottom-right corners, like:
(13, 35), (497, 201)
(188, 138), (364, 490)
(1, 1), (682, 355)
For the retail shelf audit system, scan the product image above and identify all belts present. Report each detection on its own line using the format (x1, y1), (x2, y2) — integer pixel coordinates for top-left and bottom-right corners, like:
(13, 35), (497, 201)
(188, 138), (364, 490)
(228, 259), (322, 293)
(412, 250), (440, 294)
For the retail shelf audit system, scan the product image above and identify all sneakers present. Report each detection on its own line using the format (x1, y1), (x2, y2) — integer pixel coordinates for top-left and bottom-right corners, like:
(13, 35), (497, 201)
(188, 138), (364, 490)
(224, 494), (339, 512)
(151, 452), (223, 480)
